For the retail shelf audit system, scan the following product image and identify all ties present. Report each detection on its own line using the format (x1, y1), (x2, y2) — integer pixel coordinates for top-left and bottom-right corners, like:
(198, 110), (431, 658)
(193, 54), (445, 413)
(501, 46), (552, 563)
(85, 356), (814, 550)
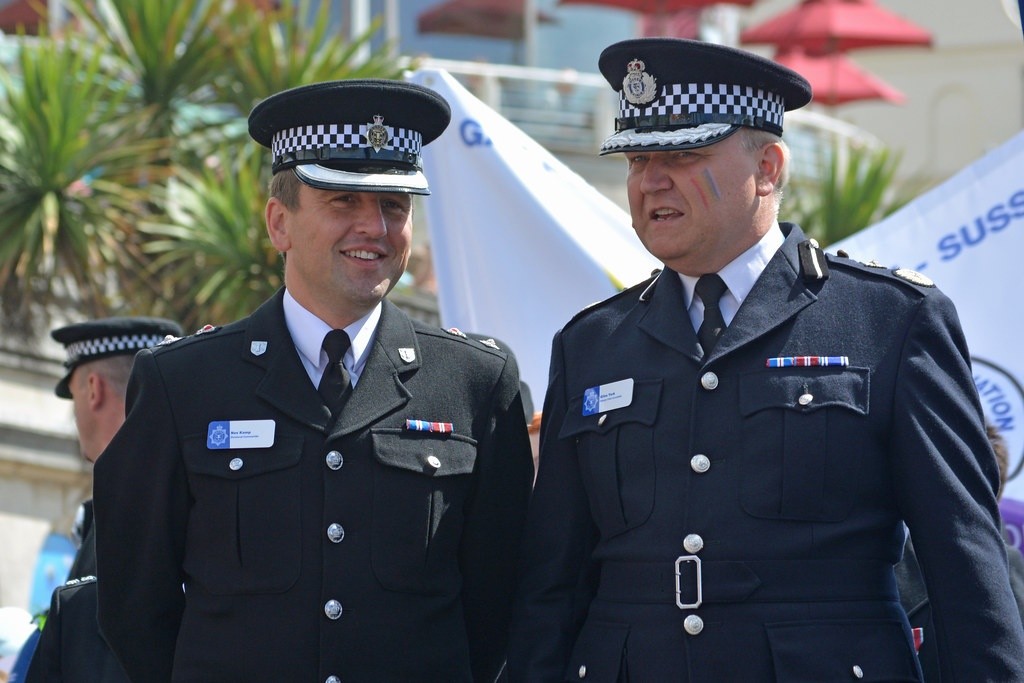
(693, 273), (727, 358)
(316, 327), (352, 417)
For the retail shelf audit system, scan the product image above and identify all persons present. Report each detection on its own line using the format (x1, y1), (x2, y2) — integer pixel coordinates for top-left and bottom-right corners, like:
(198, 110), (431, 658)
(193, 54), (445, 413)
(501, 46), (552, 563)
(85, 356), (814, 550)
(503, 34), (1024, 681)
(891, 419), (1024, 683)
(8, 315), (188, 682)
(92, 79), (541, 681)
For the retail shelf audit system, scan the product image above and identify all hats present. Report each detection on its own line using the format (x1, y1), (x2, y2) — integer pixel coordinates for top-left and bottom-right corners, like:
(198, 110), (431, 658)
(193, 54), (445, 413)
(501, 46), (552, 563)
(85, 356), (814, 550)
(247, 76), (453, 195)
(597, 37), (811, 156)
(51, 318), (184, 400)
